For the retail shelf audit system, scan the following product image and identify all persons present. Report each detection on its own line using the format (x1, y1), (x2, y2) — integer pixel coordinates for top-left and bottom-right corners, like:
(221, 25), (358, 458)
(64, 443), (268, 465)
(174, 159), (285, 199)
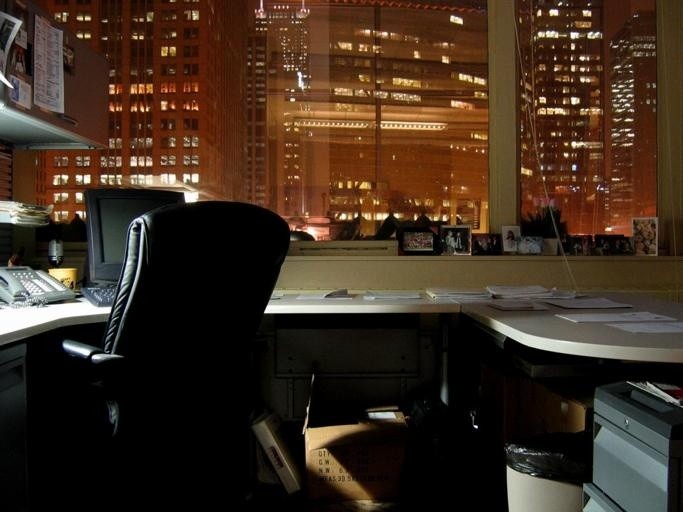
(596, 236), (625, 254)
(521, 237), (544, 254)
(471, 236), (499, 254)
(453, 231), (467, 253)
(505, 229), (520, 251)
(443, 230), (455, 257)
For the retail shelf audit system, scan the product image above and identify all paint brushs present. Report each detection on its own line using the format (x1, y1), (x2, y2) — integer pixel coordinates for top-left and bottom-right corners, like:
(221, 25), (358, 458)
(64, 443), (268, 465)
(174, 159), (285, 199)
(526, 205), (562, 223)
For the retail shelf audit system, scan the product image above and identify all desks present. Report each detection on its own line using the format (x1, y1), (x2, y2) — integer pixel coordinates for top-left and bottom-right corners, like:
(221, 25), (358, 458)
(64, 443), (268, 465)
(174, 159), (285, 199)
(1, 287), (682, 425)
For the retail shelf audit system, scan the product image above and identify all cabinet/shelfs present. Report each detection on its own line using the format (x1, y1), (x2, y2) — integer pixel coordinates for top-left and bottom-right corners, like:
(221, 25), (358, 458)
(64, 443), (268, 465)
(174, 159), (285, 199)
(0, 0), (110, 151)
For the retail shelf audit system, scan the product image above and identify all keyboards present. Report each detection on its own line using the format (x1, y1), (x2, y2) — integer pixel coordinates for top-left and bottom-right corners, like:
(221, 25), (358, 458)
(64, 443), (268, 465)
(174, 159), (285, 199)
(80, 284), (116, 307)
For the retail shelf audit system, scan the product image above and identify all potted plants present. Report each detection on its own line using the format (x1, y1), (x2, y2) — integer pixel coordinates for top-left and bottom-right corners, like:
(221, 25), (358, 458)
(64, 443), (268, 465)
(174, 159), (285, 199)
(521, 204), (569, 257)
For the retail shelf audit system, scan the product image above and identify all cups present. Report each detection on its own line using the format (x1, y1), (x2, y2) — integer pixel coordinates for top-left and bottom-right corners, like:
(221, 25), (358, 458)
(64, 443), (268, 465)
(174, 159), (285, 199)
(48, 267), (76, 292)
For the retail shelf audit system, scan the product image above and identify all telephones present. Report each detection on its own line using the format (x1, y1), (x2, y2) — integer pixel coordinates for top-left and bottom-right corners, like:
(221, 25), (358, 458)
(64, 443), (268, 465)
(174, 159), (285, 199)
(0, 266), (76, 306)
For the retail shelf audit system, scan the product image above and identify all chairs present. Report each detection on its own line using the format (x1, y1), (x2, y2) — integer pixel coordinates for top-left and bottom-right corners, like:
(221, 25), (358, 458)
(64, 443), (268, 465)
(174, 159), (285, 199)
(48, 199), (291, 512)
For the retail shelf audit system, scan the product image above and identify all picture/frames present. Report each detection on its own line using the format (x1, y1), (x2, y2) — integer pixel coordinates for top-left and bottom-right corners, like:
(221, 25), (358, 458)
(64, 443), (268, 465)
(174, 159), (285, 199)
(394, 216), (659, 257)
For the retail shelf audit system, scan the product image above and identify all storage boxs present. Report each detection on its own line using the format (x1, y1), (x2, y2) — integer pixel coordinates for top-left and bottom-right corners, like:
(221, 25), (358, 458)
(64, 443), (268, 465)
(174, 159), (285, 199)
(297, 371), (429, 511)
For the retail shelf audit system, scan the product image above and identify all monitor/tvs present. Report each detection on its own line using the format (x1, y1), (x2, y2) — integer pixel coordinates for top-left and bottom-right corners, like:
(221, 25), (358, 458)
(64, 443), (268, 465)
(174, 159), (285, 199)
(85, 188), (185, 285)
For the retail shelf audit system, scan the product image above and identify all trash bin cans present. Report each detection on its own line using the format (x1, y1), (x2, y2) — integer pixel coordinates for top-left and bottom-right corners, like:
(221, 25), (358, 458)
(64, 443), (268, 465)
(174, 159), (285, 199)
(504, 432), (592, 512)
